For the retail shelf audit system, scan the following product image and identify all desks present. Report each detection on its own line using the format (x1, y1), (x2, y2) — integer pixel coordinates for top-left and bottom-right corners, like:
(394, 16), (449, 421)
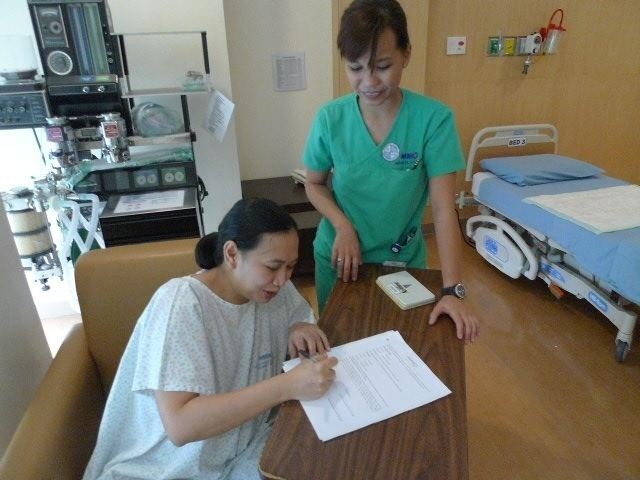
(259, 261), (470, 480)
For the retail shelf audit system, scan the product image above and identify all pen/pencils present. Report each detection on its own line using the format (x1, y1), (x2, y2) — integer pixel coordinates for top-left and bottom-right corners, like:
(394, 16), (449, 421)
(299, 350), (317, 362)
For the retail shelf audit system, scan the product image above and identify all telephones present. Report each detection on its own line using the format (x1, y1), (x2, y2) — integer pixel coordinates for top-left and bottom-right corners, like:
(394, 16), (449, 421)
(292, 169), (306, 184)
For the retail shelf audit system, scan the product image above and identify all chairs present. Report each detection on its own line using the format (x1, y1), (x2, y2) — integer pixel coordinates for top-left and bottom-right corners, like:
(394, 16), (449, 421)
(0, 236), (208, 480)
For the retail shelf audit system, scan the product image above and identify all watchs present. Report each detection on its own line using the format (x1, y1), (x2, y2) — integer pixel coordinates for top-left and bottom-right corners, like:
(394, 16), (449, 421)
(442, 283), (468, 300)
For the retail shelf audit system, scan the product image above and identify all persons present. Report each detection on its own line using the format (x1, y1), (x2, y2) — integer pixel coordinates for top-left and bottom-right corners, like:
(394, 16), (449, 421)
(302, 0), (482, 344)
(82, 198), (338, 480)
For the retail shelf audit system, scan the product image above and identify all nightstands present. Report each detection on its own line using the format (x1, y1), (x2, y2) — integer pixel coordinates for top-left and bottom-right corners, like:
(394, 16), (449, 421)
(242, 175), (333, 280)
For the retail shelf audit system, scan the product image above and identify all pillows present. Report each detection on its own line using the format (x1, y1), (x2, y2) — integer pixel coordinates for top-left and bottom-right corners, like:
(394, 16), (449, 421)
(478, 154), (605, 188)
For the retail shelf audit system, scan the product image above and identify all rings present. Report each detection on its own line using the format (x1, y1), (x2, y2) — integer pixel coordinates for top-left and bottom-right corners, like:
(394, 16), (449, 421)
(336, 258), (344, 262)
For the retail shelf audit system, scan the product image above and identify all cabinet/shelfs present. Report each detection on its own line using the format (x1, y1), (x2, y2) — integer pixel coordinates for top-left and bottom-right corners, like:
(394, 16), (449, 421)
(98, 188), (206, 247)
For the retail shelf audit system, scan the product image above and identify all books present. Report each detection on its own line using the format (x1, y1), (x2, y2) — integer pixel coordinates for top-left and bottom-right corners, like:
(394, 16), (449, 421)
(375, 269), (435, 311)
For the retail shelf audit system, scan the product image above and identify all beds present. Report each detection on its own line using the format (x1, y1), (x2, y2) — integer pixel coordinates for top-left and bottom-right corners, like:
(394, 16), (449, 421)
(462, 122), (640, 365)
